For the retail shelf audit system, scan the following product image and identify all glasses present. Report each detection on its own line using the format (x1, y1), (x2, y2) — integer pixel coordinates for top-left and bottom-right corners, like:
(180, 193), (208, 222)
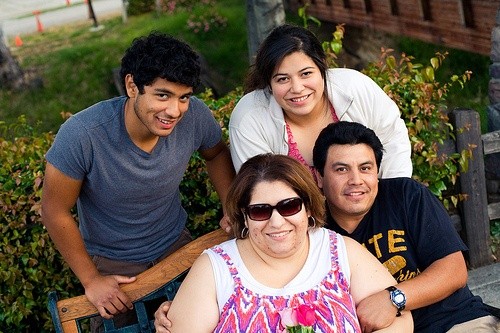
(248, 196), (302, 221)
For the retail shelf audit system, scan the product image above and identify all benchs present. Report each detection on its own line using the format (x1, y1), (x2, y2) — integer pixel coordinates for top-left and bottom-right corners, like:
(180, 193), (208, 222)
(47, 228), (230, 333)
